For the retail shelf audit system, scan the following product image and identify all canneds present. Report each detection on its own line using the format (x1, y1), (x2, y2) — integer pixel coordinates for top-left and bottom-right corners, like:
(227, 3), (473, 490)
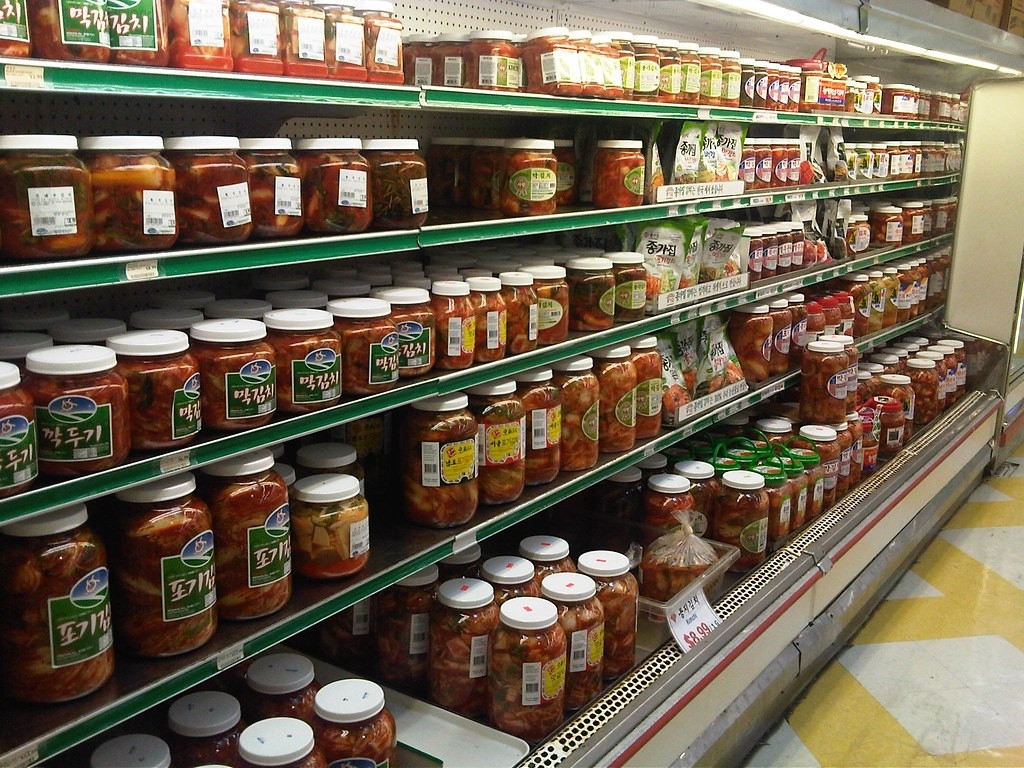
(0, 0), (996, 768)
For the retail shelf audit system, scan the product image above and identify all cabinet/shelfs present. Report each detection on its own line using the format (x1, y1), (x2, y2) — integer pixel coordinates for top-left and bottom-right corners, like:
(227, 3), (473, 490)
(0, 1), (1023, 768)
